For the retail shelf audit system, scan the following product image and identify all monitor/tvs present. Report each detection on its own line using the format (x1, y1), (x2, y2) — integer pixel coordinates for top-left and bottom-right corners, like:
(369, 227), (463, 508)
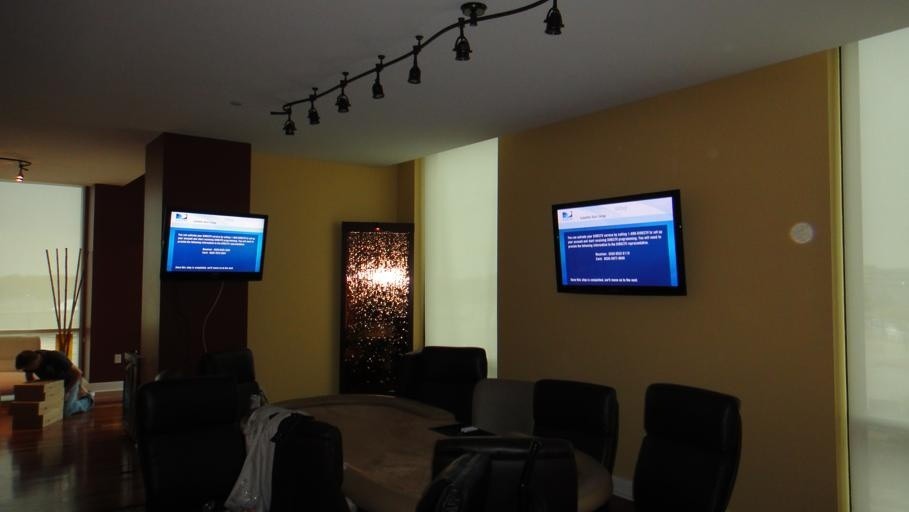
(552, 189), (687, 296)
(161, 207), (268, 281)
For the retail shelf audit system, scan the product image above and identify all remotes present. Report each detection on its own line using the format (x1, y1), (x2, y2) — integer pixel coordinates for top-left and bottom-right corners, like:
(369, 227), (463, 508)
(460, 427), (479, 433)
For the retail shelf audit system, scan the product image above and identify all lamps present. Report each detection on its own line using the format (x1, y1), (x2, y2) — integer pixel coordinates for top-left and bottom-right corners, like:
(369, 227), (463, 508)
(270, 0), (564, 135)
(0, 157), (31, 184)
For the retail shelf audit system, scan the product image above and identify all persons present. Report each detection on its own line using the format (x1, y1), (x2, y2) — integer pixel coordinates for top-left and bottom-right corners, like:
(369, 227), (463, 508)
(16, 350), (95, 418)
